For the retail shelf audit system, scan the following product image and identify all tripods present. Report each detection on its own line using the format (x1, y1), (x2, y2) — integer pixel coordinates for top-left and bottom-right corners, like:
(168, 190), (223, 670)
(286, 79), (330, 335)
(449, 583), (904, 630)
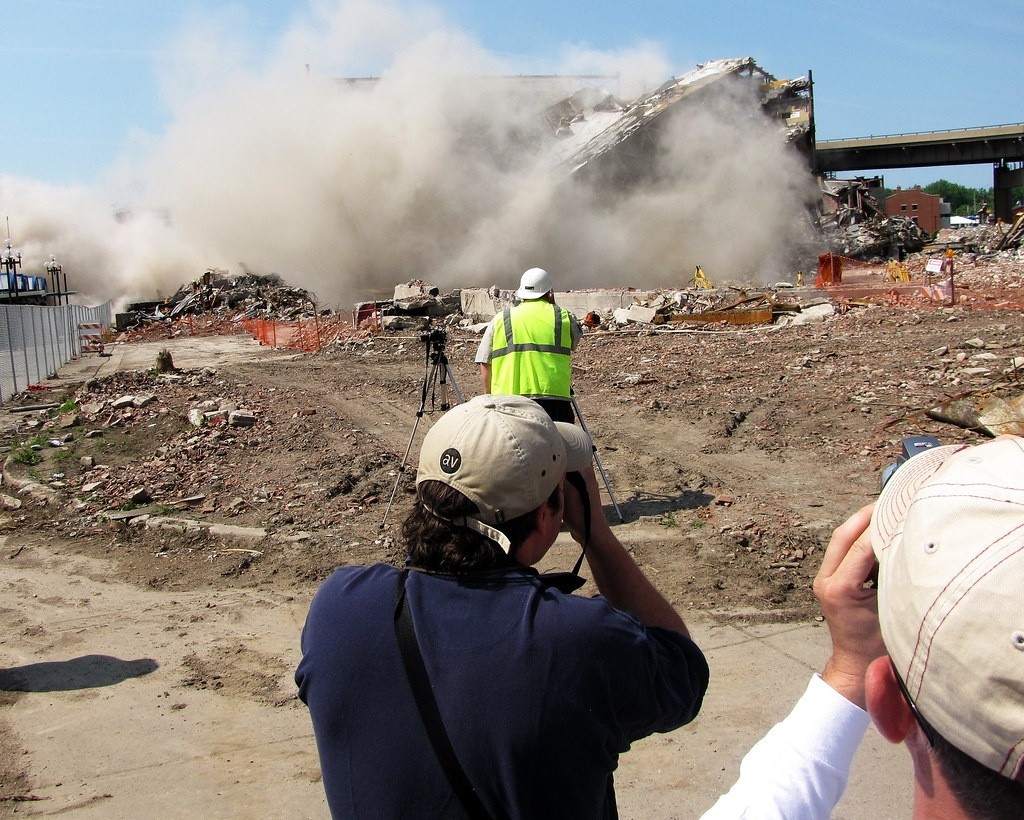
(380, 343), (463, 529)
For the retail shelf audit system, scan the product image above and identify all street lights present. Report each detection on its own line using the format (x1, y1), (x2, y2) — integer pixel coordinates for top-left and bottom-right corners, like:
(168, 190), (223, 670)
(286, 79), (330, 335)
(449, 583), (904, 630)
(45, 254), (63, 307)
(0, 238), (23, 305)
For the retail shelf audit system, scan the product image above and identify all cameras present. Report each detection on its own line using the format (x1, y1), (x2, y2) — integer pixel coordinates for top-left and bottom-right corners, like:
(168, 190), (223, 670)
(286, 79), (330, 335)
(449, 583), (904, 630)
(881, 436), (938, 492)
(420, 329), (447, 345)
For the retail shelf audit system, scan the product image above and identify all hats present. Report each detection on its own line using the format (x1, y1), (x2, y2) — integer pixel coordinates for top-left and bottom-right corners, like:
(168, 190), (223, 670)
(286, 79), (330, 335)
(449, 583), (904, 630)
(870, 434), (1024, 782)
(517, 267), (552, 300)
(415, 394), (593, 555)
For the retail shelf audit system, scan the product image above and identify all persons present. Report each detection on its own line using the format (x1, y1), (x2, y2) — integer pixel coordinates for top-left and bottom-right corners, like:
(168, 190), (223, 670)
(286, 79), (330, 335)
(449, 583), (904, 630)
(982, 203), (988, 225)
(295, 394), (711, 820)
(475, 267), (583, 426)
(698, 433), (1024, 820)
